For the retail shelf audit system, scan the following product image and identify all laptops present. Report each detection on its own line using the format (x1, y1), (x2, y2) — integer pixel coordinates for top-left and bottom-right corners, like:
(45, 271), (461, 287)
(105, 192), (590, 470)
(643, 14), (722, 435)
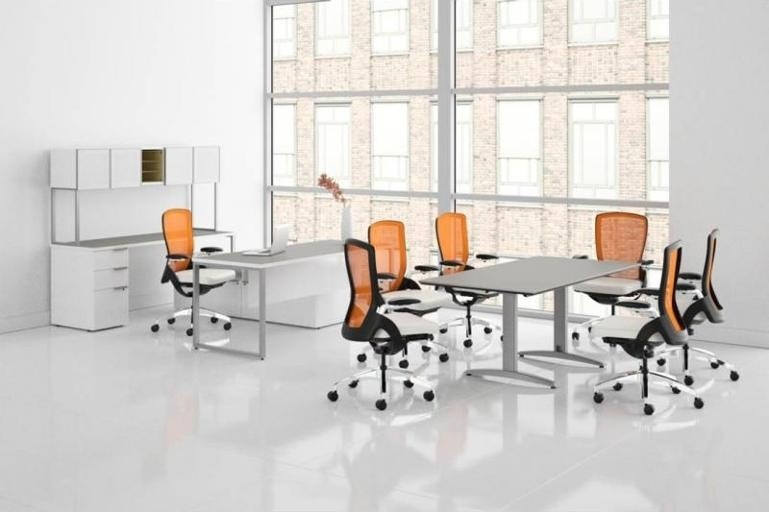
(242, 224), (289, 256)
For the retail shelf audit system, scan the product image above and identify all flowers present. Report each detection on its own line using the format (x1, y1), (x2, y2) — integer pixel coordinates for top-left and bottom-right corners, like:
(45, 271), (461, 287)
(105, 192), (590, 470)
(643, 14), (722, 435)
(317, 173), (349, 206)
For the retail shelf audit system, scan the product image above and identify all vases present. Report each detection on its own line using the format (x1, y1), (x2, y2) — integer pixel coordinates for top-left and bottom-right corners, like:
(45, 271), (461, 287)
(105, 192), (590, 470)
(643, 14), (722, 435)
(340, 202), (353, 245)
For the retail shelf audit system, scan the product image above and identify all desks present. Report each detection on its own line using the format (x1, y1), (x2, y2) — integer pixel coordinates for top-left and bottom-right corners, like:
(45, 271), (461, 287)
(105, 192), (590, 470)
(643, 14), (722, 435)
(191, 238), (370, 358)
(420, 253), (644, 388)
(51, 227), (238, 333)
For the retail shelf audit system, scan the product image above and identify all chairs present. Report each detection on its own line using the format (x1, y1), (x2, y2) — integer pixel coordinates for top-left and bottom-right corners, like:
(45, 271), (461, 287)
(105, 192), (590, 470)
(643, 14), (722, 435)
(328, 240), (436, 410)
(587, 240), (703, 415)
(570, 210), (654, 350)
(360, 220), (449, 366)
(149, 209), (242, 337)
(658, 228), (740, 385)
(436, 213), (506, 347)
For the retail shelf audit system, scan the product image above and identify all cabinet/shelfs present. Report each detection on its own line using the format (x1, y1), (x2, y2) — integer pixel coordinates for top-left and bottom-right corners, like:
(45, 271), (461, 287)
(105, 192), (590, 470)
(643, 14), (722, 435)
(48, 144), (221, 244)
